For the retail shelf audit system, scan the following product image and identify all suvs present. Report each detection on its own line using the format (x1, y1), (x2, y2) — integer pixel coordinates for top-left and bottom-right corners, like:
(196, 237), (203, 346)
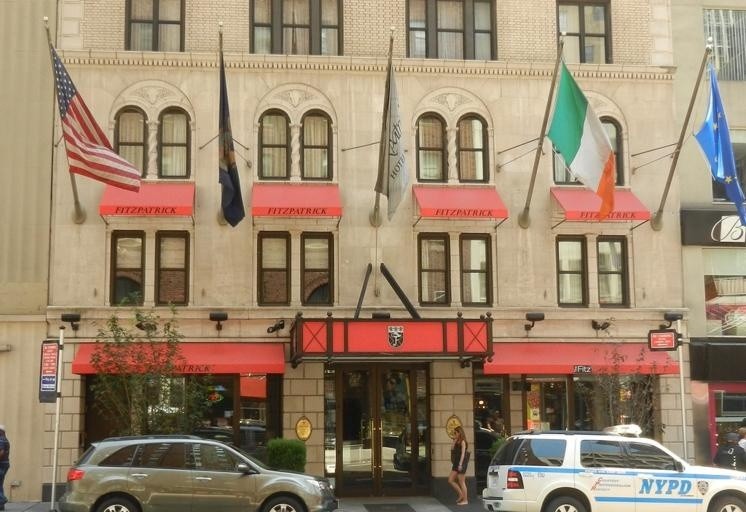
(482, 423), (746, 512)
(58, 435), (339, 511)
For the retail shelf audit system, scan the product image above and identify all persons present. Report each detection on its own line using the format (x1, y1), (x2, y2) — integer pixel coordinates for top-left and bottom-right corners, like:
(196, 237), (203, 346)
(712, 416), (746, 471)
(609, 412), (641, 436)
(475, 406), (507, 442)
(0, 424), (9, 511)
(447, 426), (469, 505)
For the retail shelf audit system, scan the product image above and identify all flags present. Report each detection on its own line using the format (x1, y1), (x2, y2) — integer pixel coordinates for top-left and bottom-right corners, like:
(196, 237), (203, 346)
(692, 63), (746, 226)
(548, 61), (617, 222)
(217, 57), (245, 227)
(48, 41), (141, 193)
(376, 63), (411, 223)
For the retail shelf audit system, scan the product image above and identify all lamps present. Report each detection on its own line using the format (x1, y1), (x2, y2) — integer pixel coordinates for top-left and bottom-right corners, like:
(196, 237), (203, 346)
(523, 312), (547, 331)
(60, 313), (82, 331)
(208, 312), (228, 332)
(660, 312), (684, 329)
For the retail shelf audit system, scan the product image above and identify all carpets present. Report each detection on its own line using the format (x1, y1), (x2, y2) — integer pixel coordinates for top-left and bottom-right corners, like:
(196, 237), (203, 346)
(363, 502), (415, 511)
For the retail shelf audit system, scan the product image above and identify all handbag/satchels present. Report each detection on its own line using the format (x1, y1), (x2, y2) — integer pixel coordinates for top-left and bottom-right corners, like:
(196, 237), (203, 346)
(451, 450), (470, 466)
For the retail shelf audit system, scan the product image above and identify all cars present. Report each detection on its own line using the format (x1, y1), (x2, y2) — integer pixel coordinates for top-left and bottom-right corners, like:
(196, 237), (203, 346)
(393, 422), (427, 470)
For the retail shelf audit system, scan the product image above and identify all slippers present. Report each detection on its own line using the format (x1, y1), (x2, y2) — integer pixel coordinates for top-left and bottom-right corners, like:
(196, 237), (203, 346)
(456, 496), (468, 505)
